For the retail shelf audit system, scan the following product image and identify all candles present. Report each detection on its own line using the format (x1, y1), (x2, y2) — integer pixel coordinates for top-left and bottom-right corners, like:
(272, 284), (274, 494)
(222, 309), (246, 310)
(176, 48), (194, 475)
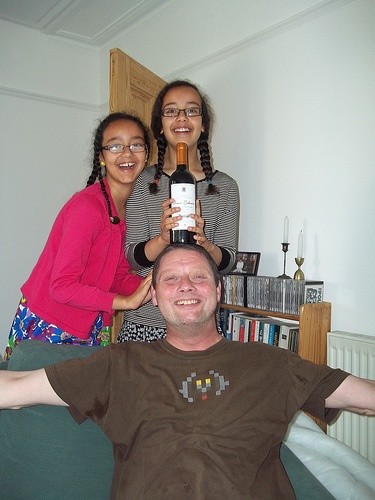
(283, 214), (289, 243)
(297, 229), (304, 258)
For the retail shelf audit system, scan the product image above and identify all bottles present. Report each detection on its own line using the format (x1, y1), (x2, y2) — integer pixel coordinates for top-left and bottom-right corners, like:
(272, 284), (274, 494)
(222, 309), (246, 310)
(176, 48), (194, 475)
(168, 142), (197, 245)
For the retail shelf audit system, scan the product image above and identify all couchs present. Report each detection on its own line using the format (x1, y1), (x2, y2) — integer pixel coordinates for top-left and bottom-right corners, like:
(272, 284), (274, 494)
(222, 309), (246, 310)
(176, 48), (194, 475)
(0, 340), (375, 500)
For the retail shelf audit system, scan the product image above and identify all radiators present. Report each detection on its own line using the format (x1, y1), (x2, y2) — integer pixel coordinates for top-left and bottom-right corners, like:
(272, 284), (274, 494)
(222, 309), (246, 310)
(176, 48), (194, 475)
(326, 330), (375, 467)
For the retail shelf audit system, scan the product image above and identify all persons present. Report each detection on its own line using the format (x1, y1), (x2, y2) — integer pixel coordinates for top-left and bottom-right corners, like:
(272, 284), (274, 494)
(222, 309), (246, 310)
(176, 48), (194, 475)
(0, 243), (375, 500)
(117, 81), (240, 343)
(5, 113), (153, 362)
(235, 259), (243, 272)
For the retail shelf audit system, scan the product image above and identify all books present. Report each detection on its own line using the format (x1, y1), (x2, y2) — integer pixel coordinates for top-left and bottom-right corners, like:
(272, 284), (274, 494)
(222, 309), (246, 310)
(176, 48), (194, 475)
(220, 309), (299, 353)
(224, 275), (324, 315)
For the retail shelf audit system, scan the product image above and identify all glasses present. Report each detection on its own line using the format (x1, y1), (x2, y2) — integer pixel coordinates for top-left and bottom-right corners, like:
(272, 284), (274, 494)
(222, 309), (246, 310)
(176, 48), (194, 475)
(160, 108), (203, 117)
(101, 143), (147, 153)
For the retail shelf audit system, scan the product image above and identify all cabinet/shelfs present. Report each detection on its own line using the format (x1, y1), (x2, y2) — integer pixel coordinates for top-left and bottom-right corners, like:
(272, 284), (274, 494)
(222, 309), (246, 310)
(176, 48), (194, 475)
(217, 301), (331, 435)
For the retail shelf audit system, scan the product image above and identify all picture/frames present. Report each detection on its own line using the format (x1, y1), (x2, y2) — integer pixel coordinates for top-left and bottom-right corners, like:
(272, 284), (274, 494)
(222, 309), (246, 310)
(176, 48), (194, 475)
(227, 252), (261, 276)
